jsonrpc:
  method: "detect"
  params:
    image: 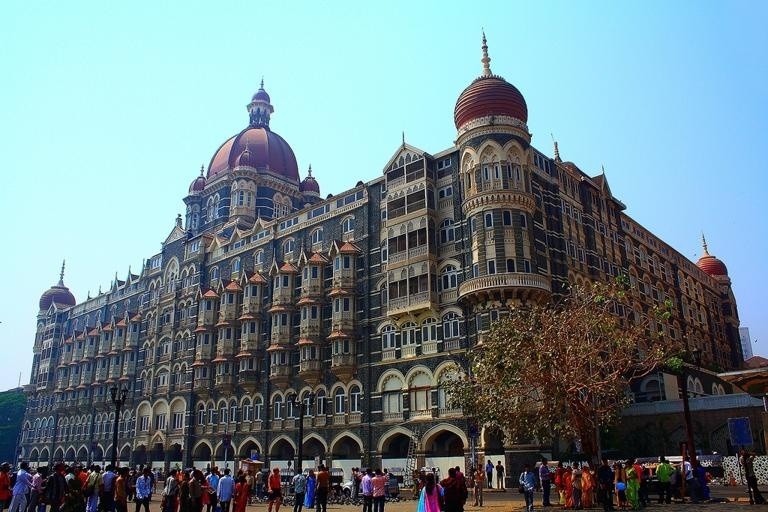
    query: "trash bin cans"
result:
[404,428,420,486]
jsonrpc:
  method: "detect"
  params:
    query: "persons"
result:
[739,447,763,505]
[349,467,390,512]
[412,466,468,512]
[517,456,711,512]
[472,464,486,506]
[485,460,505,490]
[1,459,329,512]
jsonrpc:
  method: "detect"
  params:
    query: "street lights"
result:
[658,343,702,473]
[286,390,317,476]
[109,380,130,469]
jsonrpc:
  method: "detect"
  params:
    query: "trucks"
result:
[503,449,563,490]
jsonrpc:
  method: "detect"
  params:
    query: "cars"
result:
[263,466,408,502]
